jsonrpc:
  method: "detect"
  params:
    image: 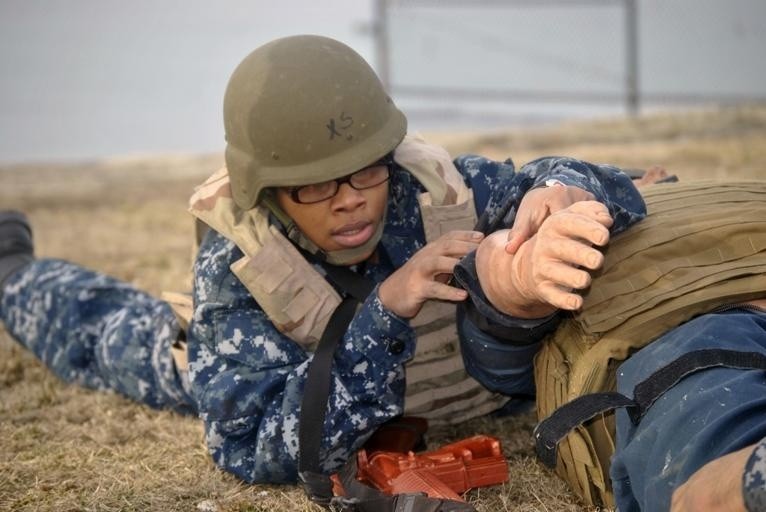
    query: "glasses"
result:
[282,154,393,204]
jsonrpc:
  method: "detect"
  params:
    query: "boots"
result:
[0,210,34,282]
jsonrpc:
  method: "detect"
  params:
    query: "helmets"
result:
[223,35,407,210]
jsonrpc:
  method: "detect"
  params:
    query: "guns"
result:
[329,435,510,503]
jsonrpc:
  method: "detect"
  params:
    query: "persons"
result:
[452,155,766,512]
[0,32,648,486]
[670,436,766,512]
